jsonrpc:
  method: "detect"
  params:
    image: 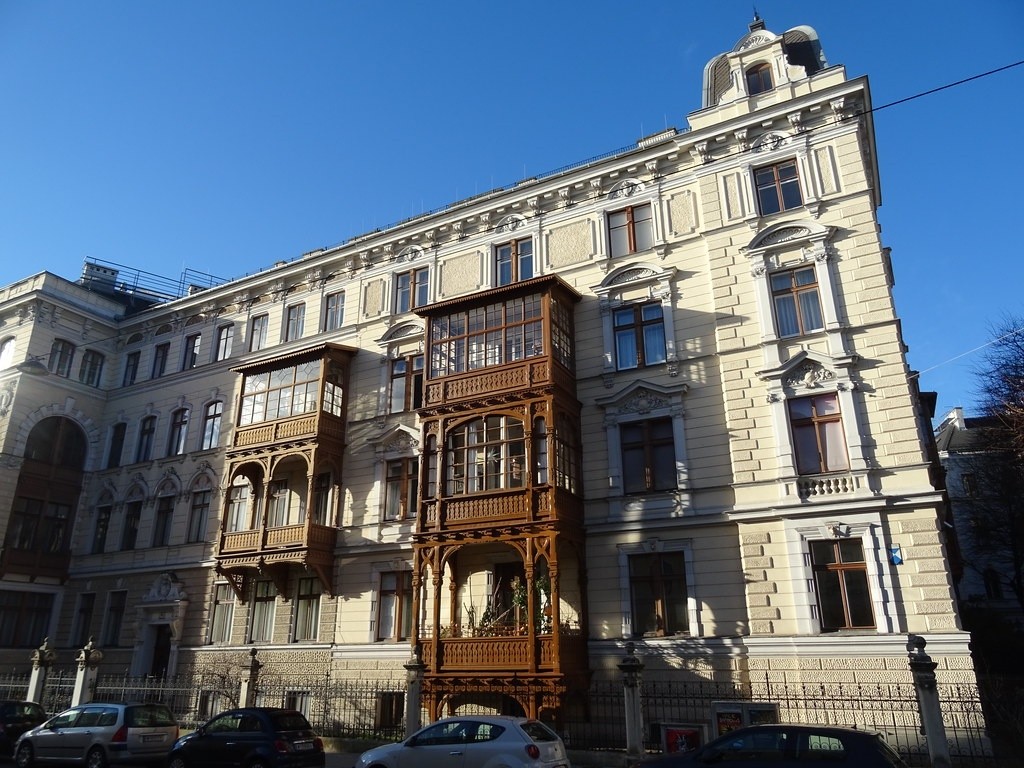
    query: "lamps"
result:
[17,354,49,375]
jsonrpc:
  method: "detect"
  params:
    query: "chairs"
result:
[239,716,257,730]
[466,725,478,739]
[78,713,102,726]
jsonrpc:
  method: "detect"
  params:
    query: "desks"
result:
[453,472,483,495]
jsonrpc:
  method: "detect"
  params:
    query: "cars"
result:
[629,721,908,768]
[0,699,49,768]
[167,706,326,767]
[13,700,179,768]
[356,715,571,768]
